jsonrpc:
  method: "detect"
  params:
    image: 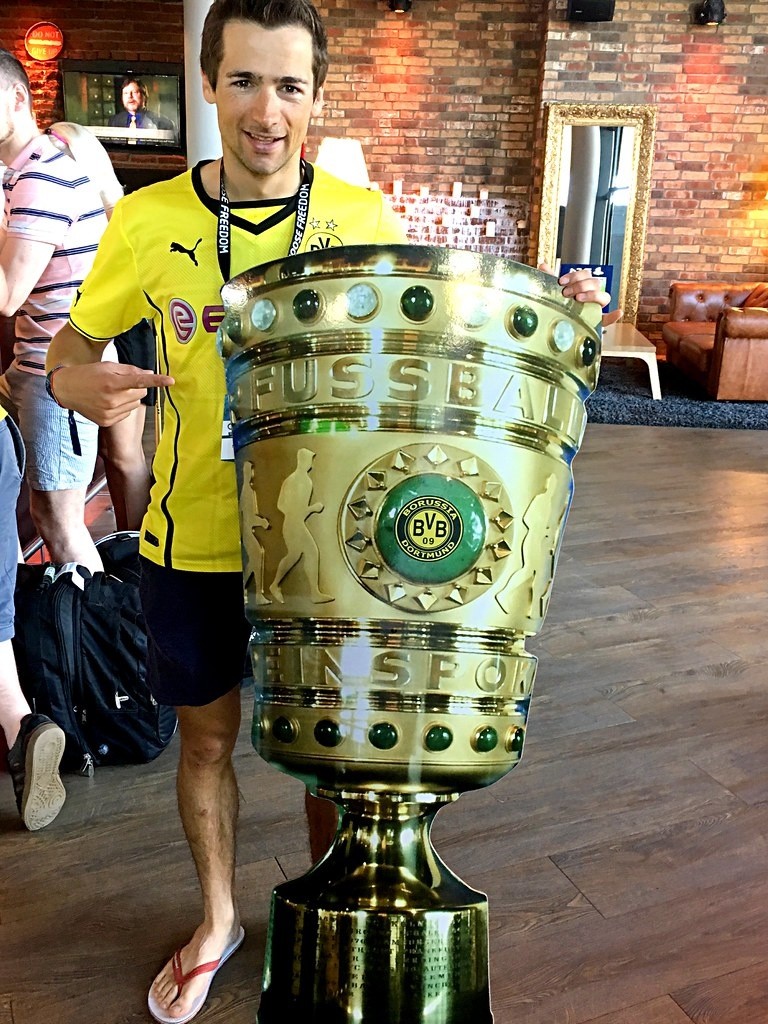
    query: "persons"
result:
[0,52,161,830]
[45,0,610,1024]
[111,75,174,145]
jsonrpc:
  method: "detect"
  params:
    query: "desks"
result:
[600,322,662,401]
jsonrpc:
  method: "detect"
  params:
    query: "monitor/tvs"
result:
[58,58,187,155]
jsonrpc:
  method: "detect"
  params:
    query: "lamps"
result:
[391,0,409,13]
[701,0,727,26]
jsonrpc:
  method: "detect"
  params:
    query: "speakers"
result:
[565,0,614,23]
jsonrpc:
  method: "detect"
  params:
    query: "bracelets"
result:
[45,365,82,457]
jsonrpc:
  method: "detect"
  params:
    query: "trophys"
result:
[221,243,604,1024]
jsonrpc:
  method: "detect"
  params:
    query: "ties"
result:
[129,117,136,128]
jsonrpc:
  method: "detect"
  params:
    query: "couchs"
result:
[663,281,768,403]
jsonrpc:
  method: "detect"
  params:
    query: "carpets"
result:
[584,356,768,431]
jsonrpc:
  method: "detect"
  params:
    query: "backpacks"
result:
[11,561,179,776]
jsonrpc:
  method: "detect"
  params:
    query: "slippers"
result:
[148,926,244,1024]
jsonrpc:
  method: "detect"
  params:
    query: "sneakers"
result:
[8,714,66,831]
